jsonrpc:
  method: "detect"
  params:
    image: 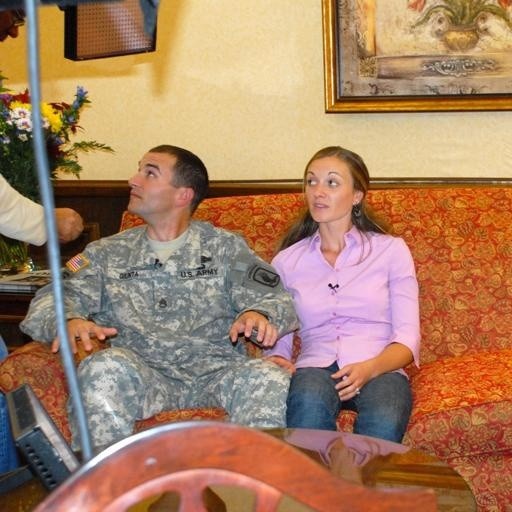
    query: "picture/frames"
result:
[322,1,512,118]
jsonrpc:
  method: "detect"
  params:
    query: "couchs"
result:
[0,181,512,511]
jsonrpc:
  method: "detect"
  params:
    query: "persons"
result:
[1,0,88,497]
[21,147,299,460]
[280,427,412,487]
[256,144,425,446]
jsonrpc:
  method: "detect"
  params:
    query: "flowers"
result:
[0,72,117,268]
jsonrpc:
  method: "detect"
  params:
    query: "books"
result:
[0,271,54,291]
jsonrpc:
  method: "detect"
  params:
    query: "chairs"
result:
[28,421,440,512]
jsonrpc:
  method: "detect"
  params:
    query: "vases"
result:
[0,234,34,277]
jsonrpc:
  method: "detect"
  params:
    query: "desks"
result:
[0,424,479,510]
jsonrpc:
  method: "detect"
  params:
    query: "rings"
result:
[354,387,361,396]
